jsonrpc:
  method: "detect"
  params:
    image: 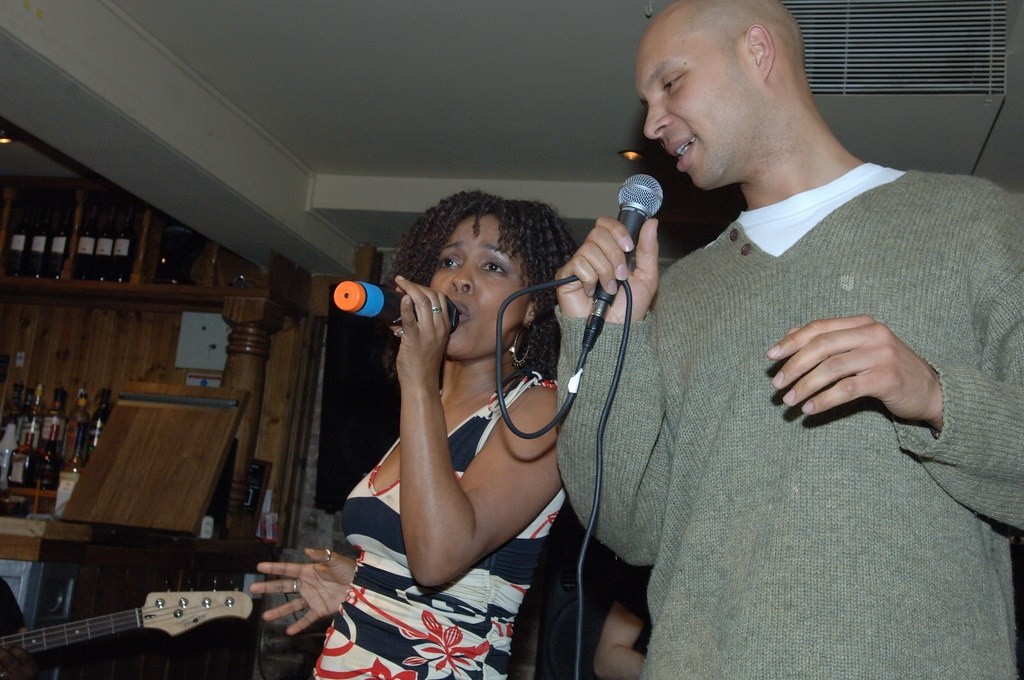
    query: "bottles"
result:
[0,380,88,486]
[8,204,135,284]
[153,217,205,285]
[87,387,112,453]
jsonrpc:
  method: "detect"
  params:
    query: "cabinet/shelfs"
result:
[0,173,312,319]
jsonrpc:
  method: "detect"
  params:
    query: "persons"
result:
[247,189,582,680]
[0,575,40,680]
[593,560,656,680]
[552,0,1024,680]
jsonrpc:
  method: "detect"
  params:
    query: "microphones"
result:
[581,174,663,349]
[334,281,459,334]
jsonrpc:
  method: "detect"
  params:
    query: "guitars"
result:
[0,575,256,662]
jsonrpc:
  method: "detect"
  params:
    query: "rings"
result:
[293,579,297,592]
[431,303,442,315]
[318,548,331,566]
[0,670,9,679]
[393,328,405,337]
